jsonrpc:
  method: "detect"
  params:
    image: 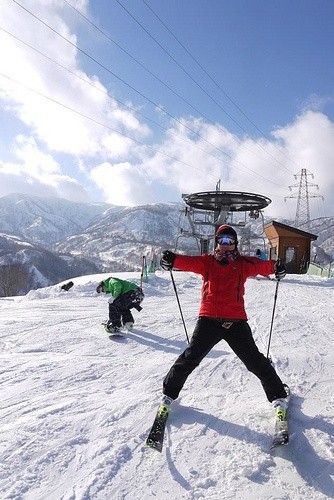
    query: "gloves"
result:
[160,251,175,270]
[274,261,286,280]
[107,296,114,303]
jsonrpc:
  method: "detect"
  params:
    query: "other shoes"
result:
[107,325,123,333]
[124,322,133,329]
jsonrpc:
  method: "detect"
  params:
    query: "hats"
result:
[215,225,236,236]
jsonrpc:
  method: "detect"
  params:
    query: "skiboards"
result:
[272,383,292,448]
[143,406,166,453]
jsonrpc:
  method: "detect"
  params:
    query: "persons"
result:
[96,276,144,334]
[156,225,287,419]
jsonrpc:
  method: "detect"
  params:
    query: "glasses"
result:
[216,235,236,244]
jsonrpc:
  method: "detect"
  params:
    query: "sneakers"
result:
[161,395,173,408]
[272,398,287,412]
